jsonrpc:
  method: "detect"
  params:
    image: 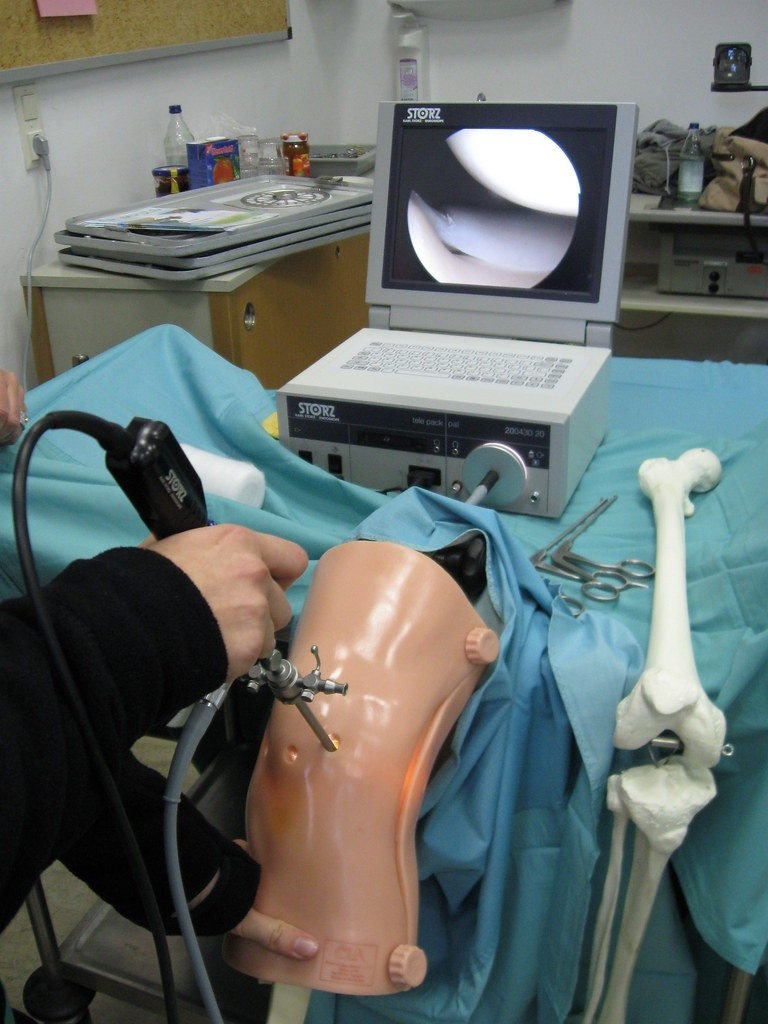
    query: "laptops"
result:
[277,104,639,423]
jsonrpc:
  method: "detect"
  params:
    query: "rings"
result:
[19,410,27,430]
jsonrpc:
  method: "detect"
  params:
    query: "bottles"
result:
[678,123,704,206]
[164,104,194,166]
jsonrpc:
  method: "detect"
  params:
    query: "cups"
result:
[236,134,286,178]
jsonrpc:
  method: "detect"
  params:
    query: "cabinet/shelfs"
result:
[619,195,768,319]
[20,170,375,391]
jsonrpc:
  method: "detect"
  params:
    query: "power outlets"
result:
[13,83,47,171]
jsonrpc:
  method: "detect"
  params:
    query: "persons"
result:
[1,369,320,1024]
